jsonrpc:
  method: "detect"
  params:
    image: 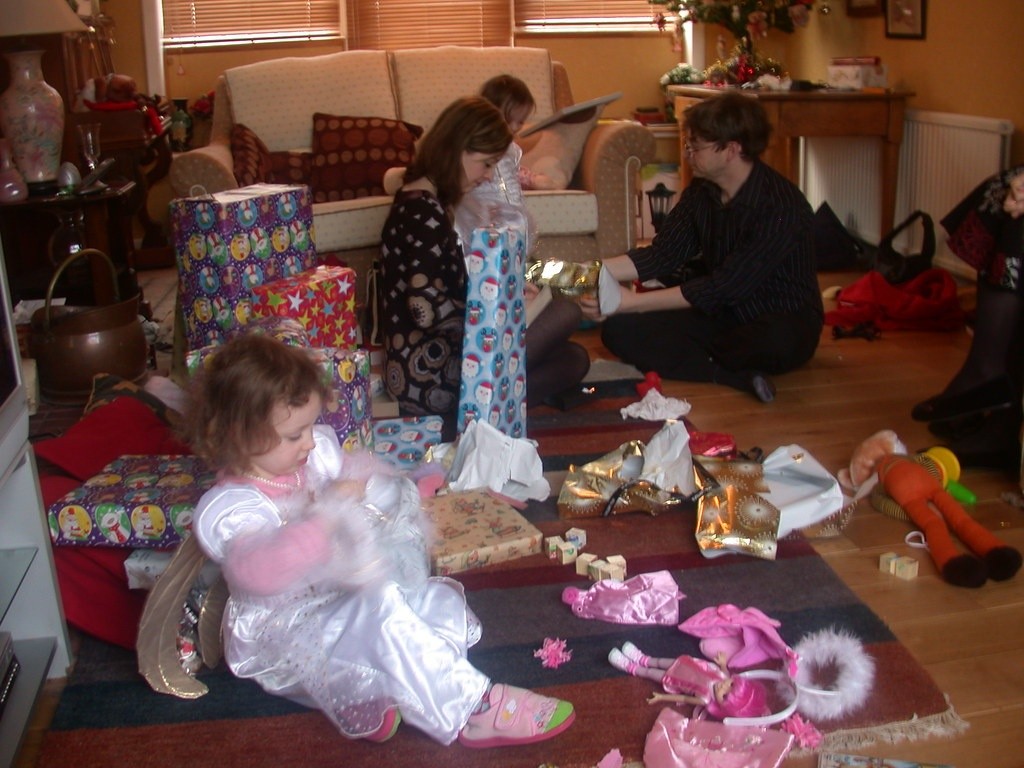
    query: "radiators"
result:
[800,108,1012,284]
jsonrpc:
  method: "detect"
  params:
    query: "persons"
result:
[911,165,1024,469]
[608,640,766,721]
[379,76,591,427]
[576,90,825,405]
[194,333,576,748]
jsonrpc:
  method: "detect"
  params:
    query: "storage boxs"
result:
[827,56,890,89]
[48,187,541,609]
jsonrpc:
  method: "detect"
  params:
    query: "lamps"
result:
[0,0,90,182]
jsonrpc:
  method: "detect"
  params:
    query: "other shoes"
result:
[458,683,577,749]
[340,698,403,744]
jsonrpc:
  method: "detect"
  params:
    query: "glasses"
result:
[684,137,718,158]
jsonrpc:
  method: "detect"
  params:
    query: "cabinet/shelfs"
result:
[0,439,76,768]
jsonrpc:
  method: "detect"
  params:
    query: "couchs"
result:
[171,47,639,300]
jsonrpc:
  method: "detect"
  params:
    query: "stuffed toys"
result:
[849,429,1024,588]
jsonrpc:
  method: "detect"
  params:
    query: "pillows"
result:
[307,112,424,202]
[231,123,312,203]
[508,104,608,190]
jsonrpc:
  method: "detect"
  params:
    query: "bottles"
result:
[169,100,192,152]
[0,140,28,202]
[0,52,65,183]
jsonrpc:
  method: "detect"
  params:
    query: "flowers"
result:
[188,91,216,146]
[649,0,818,47]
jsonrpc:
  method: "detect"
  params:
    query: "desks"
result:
[1,178,142,306]
[668,84,917,245]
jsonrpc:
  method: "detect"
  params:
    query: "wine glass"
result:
[79,123,105,187]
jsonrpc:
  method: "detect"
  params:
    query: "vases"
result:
[722,32,777,84]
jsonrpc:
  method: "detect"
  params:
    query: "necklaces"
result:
[237,467,302,492]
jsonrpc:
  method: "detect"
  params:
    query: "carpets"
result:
[30,376,964,768]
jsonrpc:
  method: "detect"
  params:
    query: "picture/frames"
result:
[885,0,929,39]
